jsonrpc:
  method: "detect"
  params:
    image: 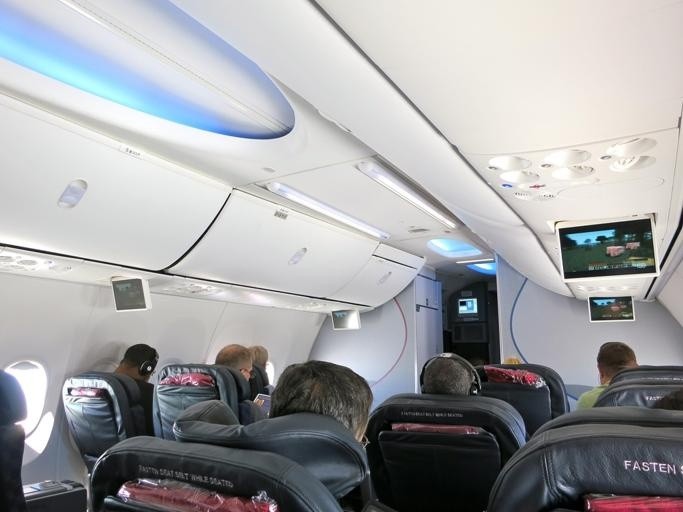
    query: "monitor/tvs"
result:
[111,277,151,312]
[556,214,661,282]
[331,310,361,330]
[588,296,635,322]
[458,299,477,314]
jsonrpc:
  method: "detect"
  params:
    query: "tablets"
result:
[254,393,270,416]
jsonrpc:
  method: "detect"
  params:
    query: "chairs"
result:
[487,407,683,512]
[89,400,371,512]
[227,365,251,400]
[592,377,683,408]
[248,363,269,395]
[152,364,255,440]
[0,370,27,512]
[62,371,146,475]
[362,393,526,512]
[474,364,570,436]
[607,366,683,385]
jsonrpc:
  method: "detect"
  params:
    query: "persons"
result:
[575,341,639,409]
[215,344,268,425]
[250,346,275,395]
[269,361,374,444]
[113,344,159,437]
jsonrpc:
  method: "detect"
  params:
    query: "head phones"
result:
[138,348,159,376]
